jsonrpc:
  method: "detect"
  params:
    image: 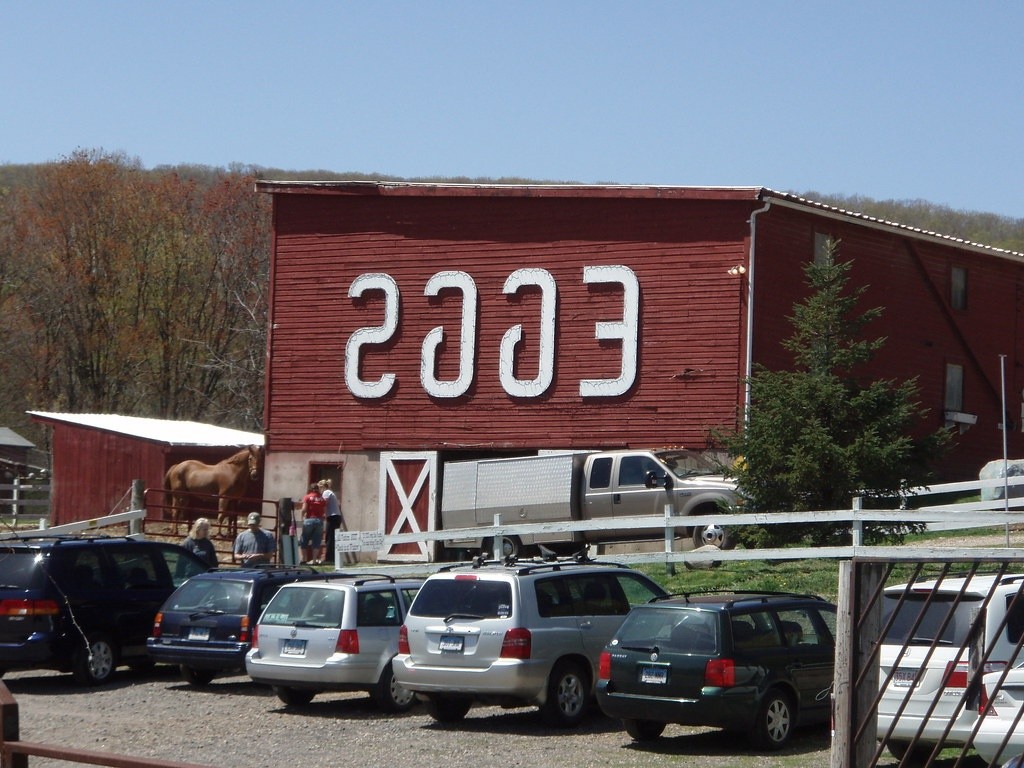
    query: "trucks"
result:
[441,442,755,564]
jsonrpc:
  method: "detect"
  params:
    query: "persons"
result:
[299,479,342,564]
[175,518,220,580]
[234,512,277,567]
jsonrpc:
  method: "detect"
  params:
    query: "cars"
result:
[971,663,1024,766]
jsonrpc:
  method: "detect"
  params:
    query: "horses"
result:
[161,446,262,539]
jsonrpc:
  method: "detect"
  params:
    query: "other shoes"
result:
[321,560,331,565]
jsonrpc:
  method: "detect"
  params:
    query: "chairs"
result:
[733,621,754,649]
[779,620,803,647]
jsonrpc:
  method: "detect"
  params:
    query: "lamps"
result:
[727,263,747,275]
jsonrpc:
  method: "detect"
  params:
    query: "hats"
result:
[309,483,319,491]
[247,512,260,526]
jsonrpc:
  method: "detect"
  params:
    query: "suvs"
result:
[592,589,838,749]
[875,571,1024,765]
[0,533,242,689]
[146,561,358,695]
[243,570,427,713]
[391,550,676,729]
